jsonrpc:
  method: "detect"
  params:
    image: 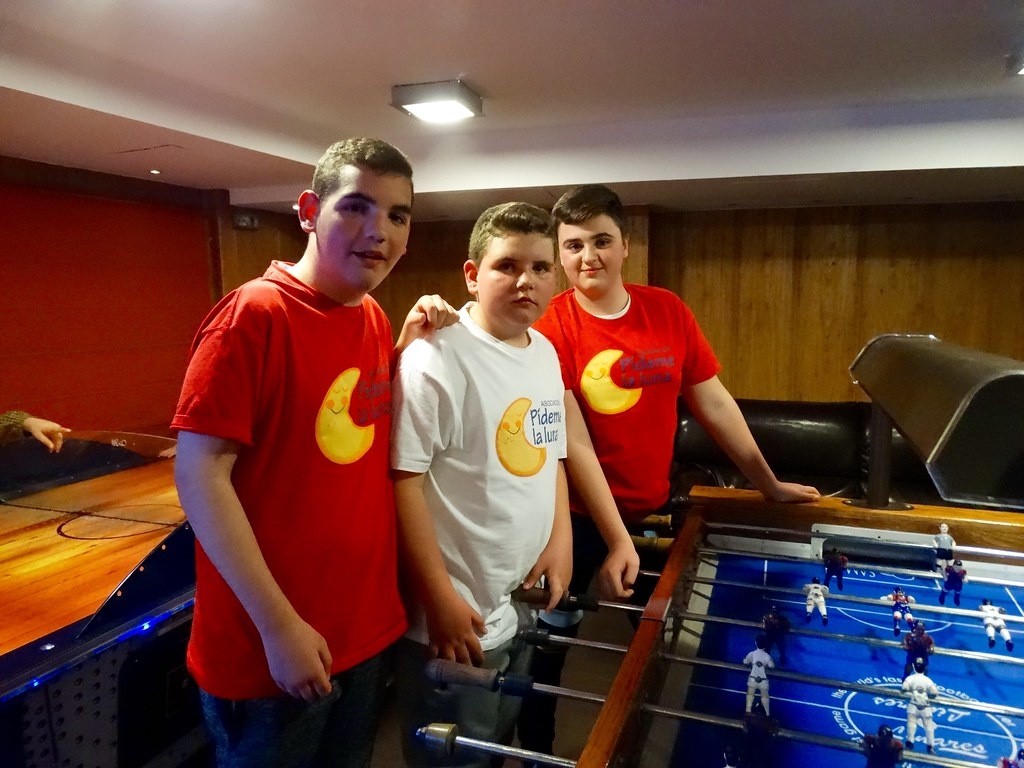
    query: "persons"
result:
[170,137,458,768]
[1,410,72,452]
[517,185,820,768]
[743,523,1024,768]
[391,204,573,768]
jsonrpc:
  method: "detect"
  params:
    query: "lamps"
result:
[388,80,484,127]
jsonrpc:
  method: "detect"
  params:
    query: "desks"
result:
[0,431,196,768]
